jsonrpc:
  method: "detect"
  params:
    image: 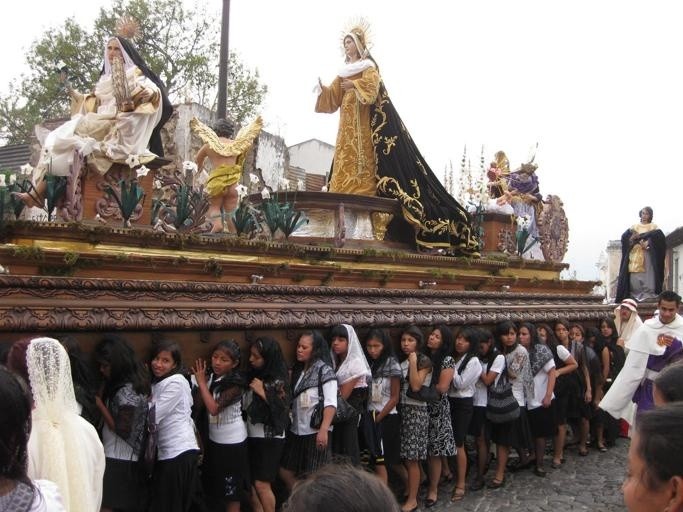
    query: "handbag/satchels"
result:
[485,383,521,423]
[406,381,440,401]
[310,363,358,429]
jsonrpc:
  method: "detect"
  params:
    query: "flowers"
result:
[0,156,307,243]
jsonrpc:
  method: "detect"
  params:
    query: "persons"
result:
[487,149,511,196]
[615,206,667,303]
[9,33,175,209]
[597,291,683,512]
[189,116,264,233]
[500,160,544,261]
[310,19,382,197]
[1,324,404,511]
[399,318,628,510]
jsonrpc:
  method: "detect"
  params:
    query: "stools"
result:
[470,211,519,255]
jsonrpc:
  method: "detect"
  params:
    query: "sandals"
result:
[399,441,609,512]
